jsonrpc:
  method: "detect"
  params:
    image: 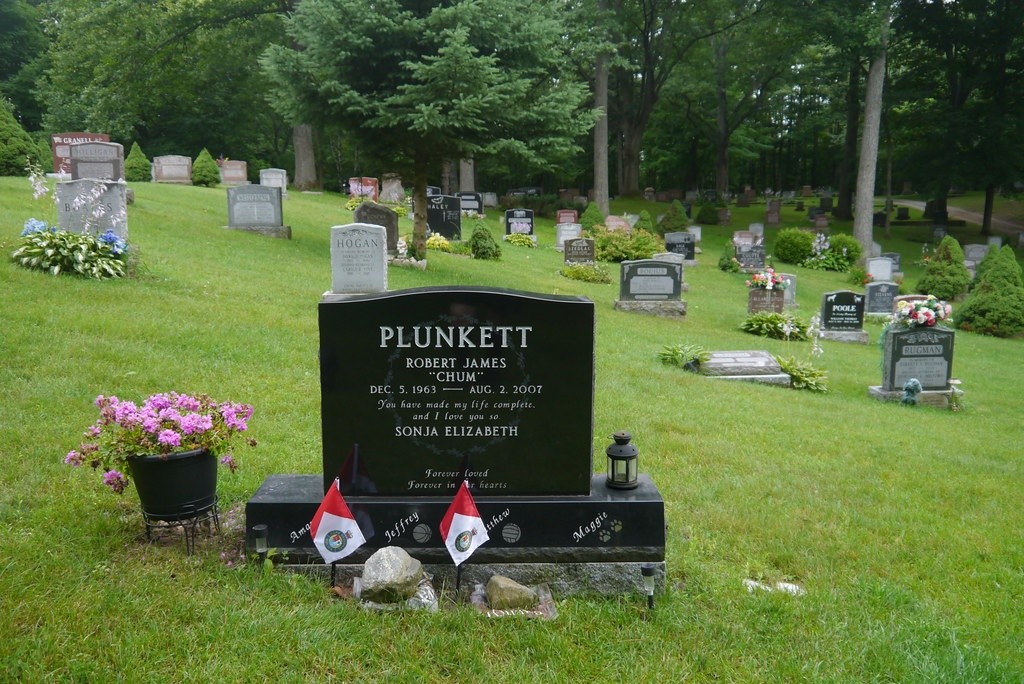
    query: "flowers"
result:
[64,391,261,493]
[9,154,129,279]
[745,266,792,291]
[731,257,745,267]
[425,232,450,250]
[810,232,830,260]
[397,235,415,259]
[882,294,955,329]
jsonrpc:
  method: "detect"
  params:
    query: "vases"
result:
[123,446,220,523]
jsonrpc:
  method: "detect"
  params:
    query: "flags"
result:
[439,478,490,567]
[310,476,368,565]
